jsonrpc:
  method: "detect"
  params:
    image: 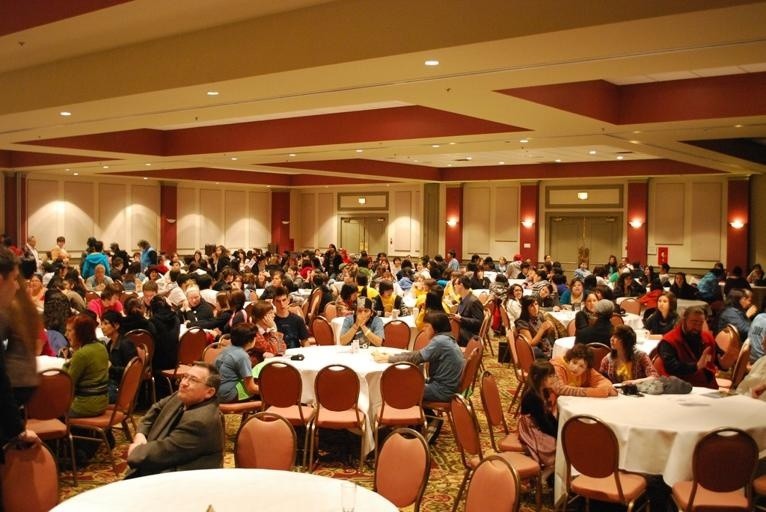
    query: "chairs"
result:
[234,412,297,471]
[451,393,542,512]
[373,361,432,466]
[71,359,142,482]
[310,361,366,471]
[465,456,520,512]
[371,428,433,512]
[671,427,760,512]
[561,415,655,512]
[479,370,535,453]
[259,361,311,473]
[1,441,60,512]
[24,370,72,480]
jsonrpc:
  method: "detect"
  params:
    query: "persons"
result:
[19,234,766,496]
[0,248,43,448]
[0,272,41,411]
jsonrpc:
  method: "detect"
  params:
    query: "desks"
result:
[556,383,766,512]
[53,468,397,512]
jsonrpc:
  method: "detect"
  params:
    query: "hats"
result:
[357,298,374,309]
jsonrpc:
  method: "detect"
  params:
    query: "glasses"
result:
[181,374,206,384]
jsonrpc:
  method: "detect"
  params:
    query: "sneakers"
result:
[536,471,549,494]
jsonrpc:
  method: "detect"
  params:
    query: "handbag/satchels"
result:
[498,341,511,363]
[614,376,693,394]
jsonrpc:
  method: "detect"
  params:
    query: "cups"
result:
[351,339,360,352]
